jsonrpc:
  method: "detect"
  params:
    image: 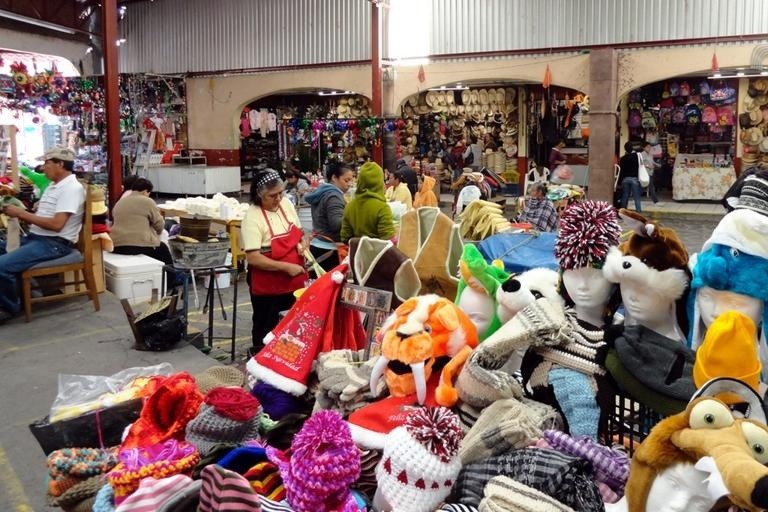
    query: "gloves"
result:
[315,347,386,409]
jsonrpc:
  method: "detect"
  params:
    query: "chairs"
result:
[22,181,102,320]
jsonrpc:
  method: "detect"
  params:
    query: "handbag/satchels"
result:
[636,152,650,188]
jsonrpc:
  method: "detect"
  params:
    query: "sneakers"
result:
[655,201,666,207]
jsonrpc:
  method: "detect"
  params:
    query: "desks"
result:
[160,262,239,367]
[158,205,244,270]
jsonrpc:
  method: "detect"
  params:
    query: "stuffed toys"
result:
[495,268,565,325]
[624,399,768,512]
[369,293,477,408]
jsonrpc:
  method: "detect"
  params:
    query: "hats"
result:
[42,438,203,512]
[244,271,345,398]
[185,386,263,460]
[35,147,75,162]
[374,404,464,511]
[738,77,768,169]
[464,384,628,510]
[400,88,517,175]
[192,410,370,512]
[693,309,763,407]
[336,95,370,118]
[90,190,115,252]
[256,259,369,368]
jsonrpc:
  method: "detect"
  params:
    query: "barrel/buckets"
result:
[179,213,213,237]
[298,207,314,230]
[203,252,234,290]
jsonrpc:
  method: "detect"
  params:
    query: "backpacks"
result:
[627,81,738,158]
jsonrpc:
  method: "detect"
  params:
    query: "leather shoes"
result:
[0,310,24,325]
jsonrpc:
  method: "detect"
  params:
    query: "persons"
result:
[278,156,438,246]
[639,142,663,207]
[561,264,613,329]
[239,168,310,360]
[698,286,765,362]
[616,141,641,213]
[549,138,568,172]
[510,182,558,231]
[459,284,495,338]
[620,280,686,349]
[0,148,174,323]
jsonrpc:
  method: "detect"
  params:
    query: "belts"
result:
[28,232,74,248]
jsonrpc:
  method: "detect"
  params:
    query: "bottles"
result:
[7,218,20,253]
